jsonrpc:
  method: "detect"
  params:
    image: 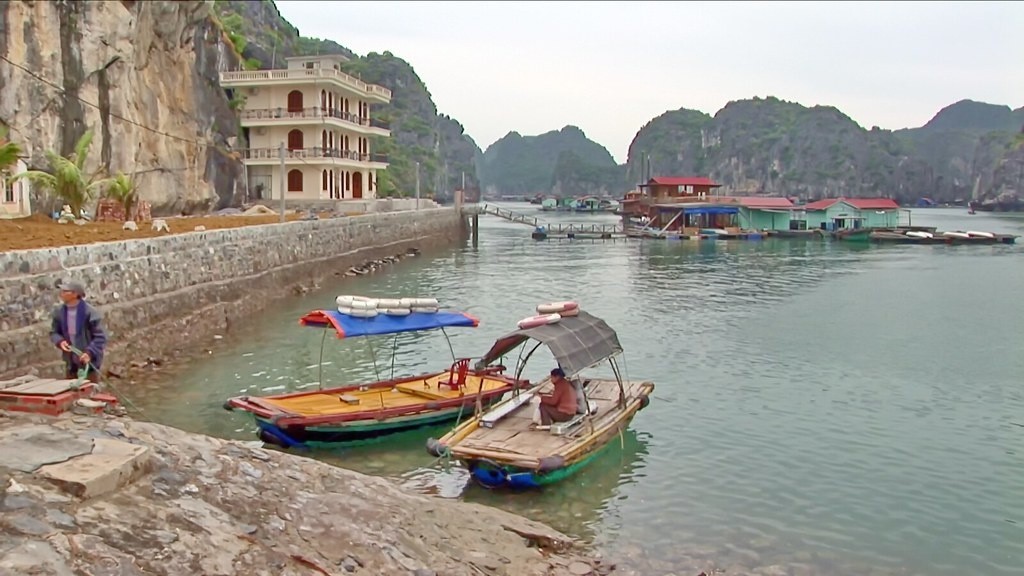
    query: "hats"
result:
[58,281,85,294]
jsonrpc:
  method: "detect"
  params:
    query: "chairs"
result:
[439,358,469,389]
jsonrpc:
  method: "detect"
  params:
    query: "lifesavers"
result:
[336,295,378,318]
[517,313,562,328]
[536,300,579,317]
[403,298,438,314]
[375,298,411,316]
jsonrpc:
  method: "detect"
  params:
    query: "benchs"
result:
[480,393,533,427]
[339,396,359,405]
[551,401,596,436]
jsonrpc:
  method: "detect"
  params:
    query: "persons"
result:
[48,280,106,384]
[536,369,578,430]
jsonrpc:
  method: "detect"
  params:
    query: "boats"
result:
[425,301,656,491]
[832,216,871,241]
[221,293,531,445]
[528,194,623,214]
